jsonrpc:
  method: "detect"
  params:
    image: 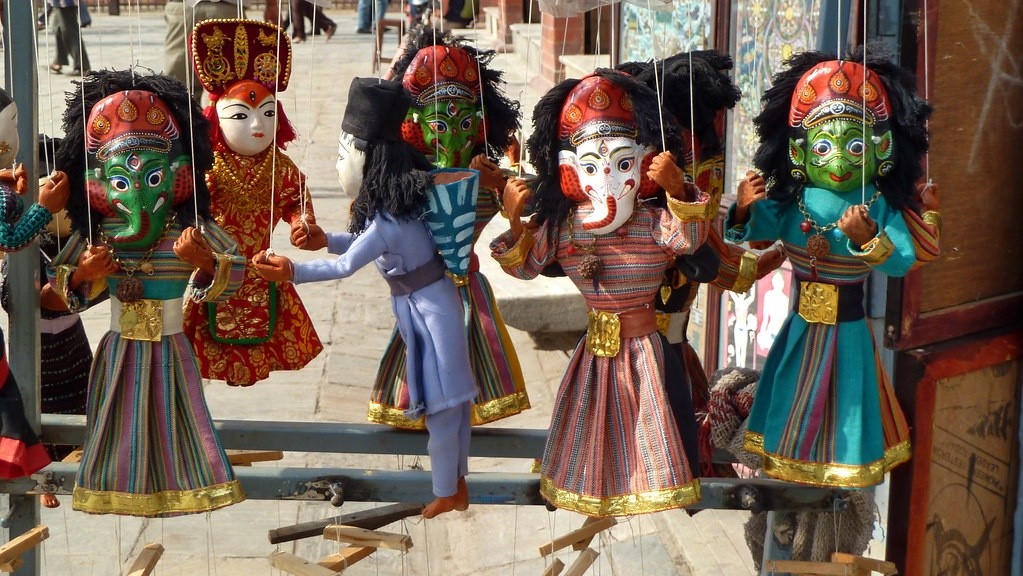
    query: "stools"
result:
[372,18,406,74]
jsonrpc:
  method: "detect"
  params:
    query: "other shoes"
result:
[374,27,392,33]
[307,30,320,35]
[357,28,371,34]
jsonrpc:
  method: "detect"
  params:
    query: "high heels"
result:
[325,26,336,40]
[292,35,306,43]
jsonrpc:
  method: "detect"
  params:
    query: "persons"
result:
[489,68,711,518]
[251,77,482,518]
[38,0,92,77]
[863,174,942,477]
[178,19,325,387]
[163,0,251,111]
[258,0,479,45]
[610,49,788,518]
[0,132,115,508]
[368,27,538,431]
[0,85,71,480]
[45,66,246,519]
[724,48,934,490]
